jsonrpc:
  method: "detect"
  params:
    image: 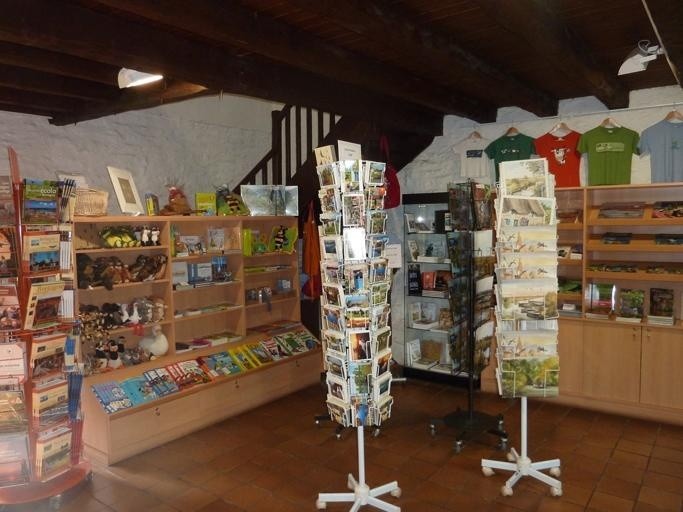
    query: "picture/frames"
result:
[107,166,145,213]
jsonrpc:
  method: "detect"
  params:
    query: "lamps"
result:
[118,67,164,90]
[618,39,662,76]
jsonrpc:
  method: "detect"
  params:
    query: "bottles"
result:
[134,226,160,246]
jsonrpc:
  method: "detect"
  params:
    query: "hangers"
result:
[446,103,683,150]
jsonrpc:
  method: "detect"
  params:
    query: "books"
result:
[406,181,496,377]
[2,175,85,486]
[92,350,242,415]
[493,158,560,396]
[314,138,393,428]
[230,327,320,370]
[174,226,301,352]
[557,201,681,325]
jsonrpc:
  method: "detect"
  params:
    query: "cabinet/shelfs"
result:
[66,215,323,466]
[402,192,480,388]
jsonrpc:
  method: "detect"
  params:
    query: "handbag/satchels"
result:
[303,220,321,277]
[385,164,400,210]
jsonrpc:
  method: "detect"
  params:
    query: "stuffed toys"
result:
[76,224,169,373]
